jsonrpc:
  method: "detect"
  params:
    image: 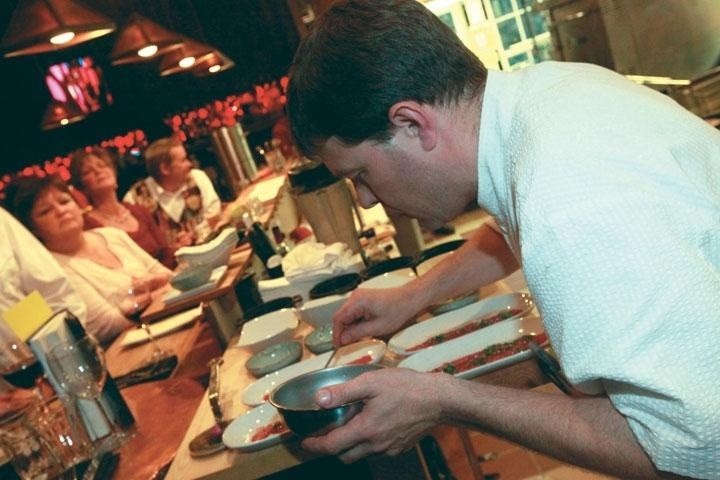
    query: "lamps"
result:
[0,0,236,79]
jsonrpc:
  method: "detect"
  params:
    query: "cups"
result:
[284,158,363,260]
[0,397,80,480]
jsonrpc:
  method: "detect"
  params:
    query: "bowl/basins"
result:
[169,264,212,292]
[174,227,239,266]
[268,364,386,435]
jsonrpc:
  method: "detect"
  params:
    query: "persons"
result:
[1,138,224,389]
[286,0,720,480]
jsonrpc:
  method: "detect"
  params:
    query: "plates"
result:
[358,266,417,295]
[416,250,459,281]
[386,292,535,357]
[160,265,228,304]
[299,289,353,327]
[395,315,551,380]
[428,291,482,313]
[303,322,336,350]
[233,308,297,348]
[120,302,203,346]
[245,343,303,376]
[222,402,290,452]
[242,339,386,405]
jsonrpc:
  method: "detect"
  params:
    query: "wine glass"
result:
[0,333,139,452]
[112,276,173,363]
